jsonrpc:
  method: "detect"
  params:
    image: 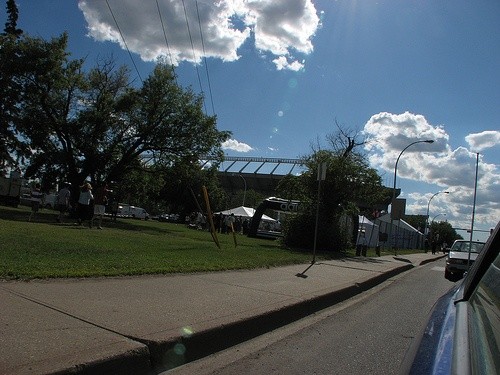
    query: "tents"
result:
[354,214,380,246]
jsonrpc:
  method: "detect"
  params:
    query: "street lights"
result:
[425,191,450,253]
[387,138,434,254]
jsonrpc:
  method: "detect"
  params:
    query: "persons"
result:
[55,182,71,224]
[159,204,283,238]
[88,180,113,231]
[28,182,44,221]
[74,182,95,226]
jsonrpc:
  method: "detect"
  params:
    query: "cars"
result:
[444,239,487,278]
